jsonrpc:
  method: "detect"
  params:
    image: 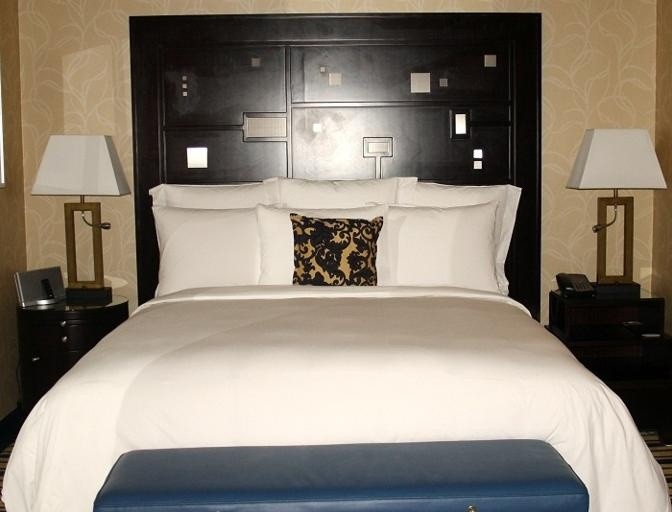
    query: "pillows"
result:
[263,176,418,209]
[413,182,522,296]
[256,203,390,285]
[290,213,383,286]
[365,198,501,295]
[149,183,266,209]
[151,203,283,299]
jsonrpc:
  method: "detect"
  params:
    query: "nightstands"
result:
[13,296,132,416]
[548,289,668,437]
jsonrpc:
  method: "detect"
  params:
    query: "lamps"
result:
[29,130,132,305]
[565,122,669,285]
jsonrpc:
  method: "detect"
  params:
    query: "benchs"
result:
[92,437,590,512]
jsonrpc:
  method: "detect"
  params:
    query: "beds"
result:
[2,290,667,511]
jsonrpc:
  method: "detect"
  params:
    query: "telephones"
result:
[556,273,595,295]
